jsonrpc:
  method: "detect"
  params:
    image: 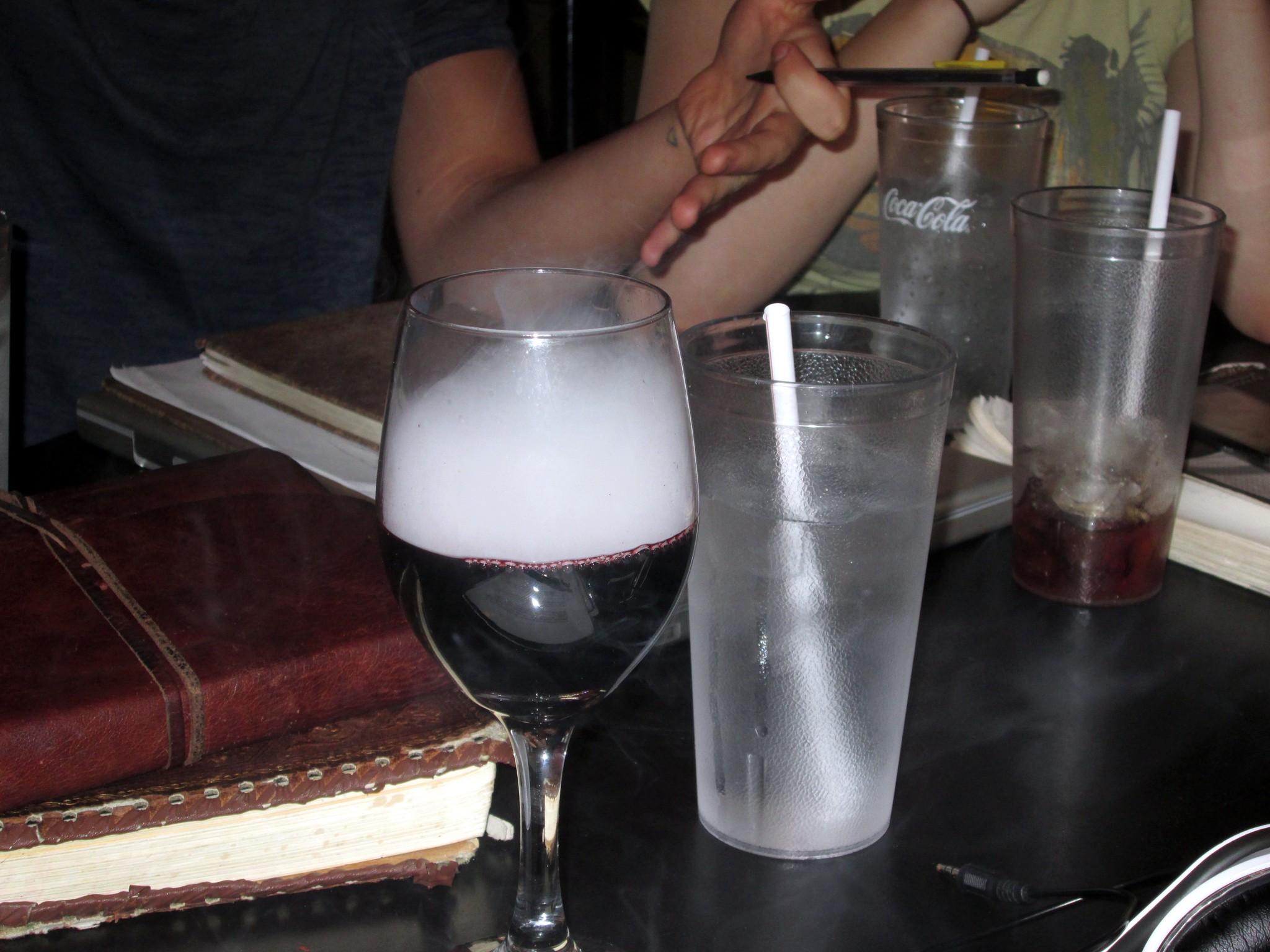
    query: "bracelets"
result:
[953,0,975,31]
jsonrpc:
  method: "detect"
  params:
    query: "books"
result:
[0,299,516,941]
[928,362,1270,599]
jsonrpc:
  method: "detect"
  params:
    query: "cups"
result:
[876,95,1048,432]
[1010,184,1227,607]
[676,313,960,856]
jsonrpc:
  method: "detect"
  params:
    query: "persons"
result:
[619,0,1270,344]
[0,0,852,460]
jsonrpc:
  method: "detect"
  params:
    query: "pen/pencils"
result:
[743,67,1049,86]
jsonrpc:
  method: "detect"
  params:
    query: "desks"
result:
[1,436,1270,952]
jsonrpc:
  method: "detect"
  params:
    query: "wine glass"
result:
[375,264,698,952]
